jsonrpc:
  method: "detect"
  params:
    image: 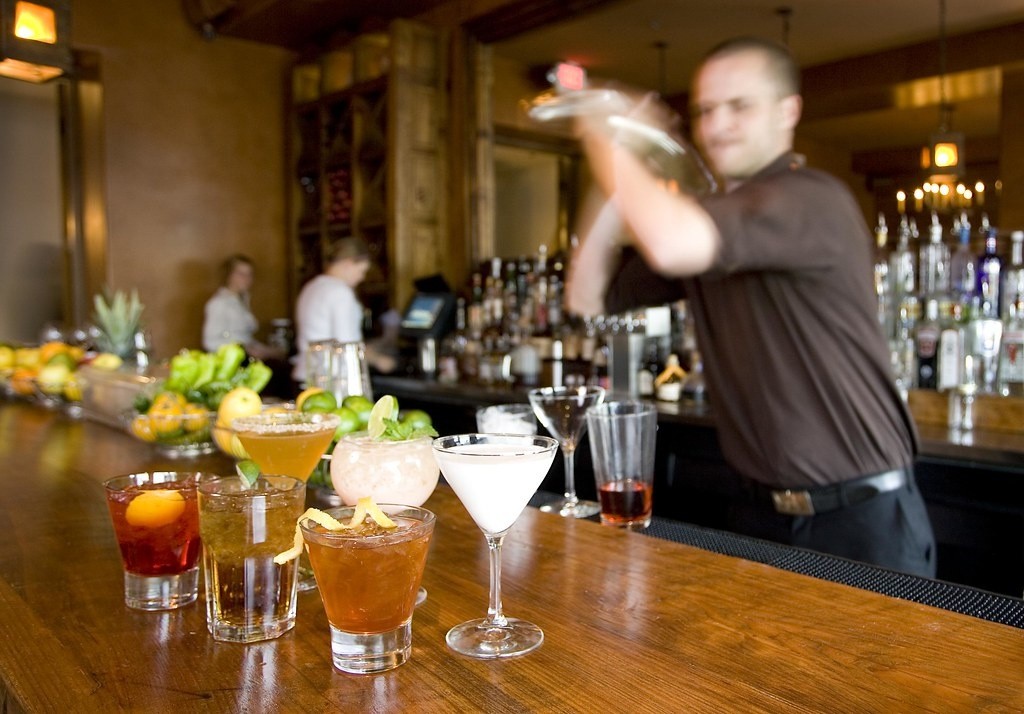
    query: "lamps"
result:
[0,0,73,87]
[920,0,967,184]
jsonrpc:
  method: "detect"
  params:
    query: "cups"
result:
[473,404,538,444]
[103,471,208,609]
[195,472,307,644]
[298,501,438,673]
[580,400,654,533]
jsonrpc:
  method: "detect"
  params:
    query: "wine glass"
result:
[432,430,561,657]
[525,384,605,519]
[329,436,440,608]
[233,411,339,590]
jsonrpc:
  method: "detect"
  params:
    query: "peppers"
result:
[131,343,271,408]
[156,429,210,447]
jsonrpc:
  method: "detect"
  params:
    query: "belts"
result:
[732,466,910,517]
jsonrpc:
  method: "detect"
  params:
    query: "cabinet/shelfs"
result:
[287,17,438,323]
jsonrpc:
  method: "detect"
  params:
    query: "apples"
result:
[211,388,327,457]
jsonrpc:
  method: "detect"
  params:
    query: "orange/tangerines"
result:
[0,339,84,398]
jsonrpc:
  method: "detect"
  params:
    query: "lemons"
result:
[235,459,262,490]
[126,489,185,526]
[302,390,435,489]
[131,391,209,442]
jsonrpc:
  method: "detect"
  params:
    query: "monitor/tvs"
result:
[399,291,459,341]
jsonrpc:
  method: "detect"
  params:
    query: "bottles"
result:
[453,190,1024,431]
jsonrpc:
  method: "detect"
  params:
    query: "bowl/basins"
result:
[123,410,269,459]
[305,428,441,508]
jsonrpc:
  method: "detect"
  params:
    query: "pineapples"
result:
[64,290,143,403]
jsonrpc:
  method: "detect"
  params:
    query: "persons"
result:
[295,236,395,408]
[199,253,277,368]
[564,36,935,579]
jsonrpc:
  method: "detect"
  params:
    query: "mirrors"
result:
[446,0,1024,254]
